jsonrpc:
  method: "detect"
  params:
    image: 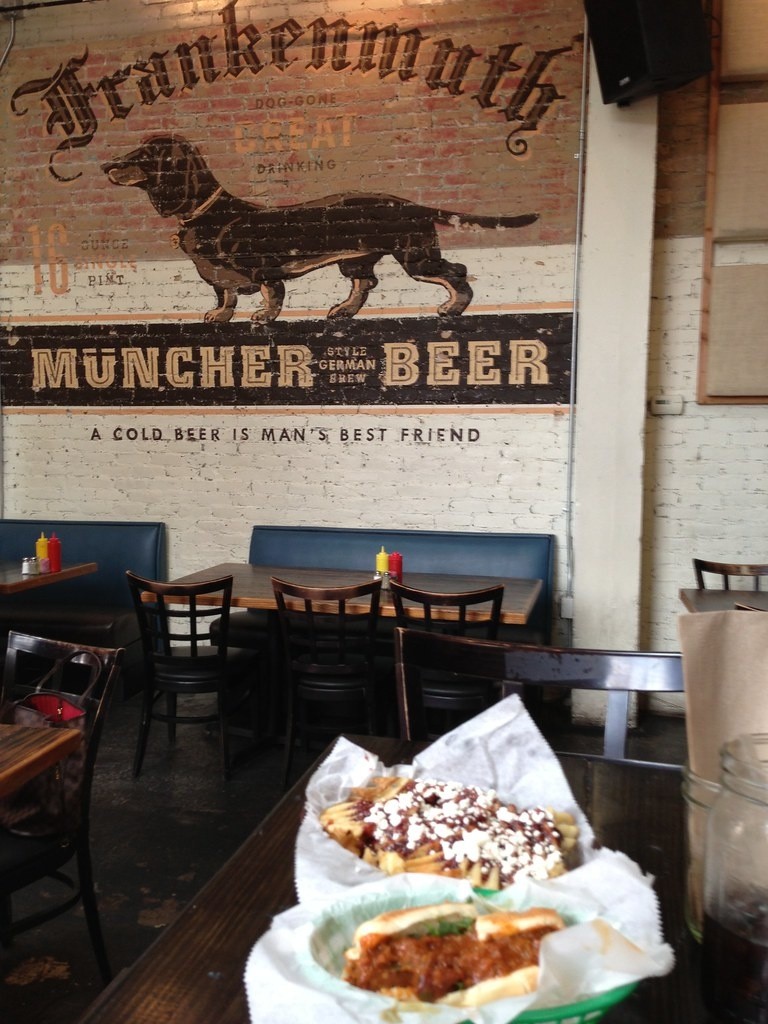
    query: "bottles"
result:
[376,545,388,577]
[373,571,382,580]
[35,531,48,561]
[47,532,61,572]
[21,557,30,574]
[30,557,39,575]
[381,573,390,589]
[388,552,402,584]
[701,732,768,1024]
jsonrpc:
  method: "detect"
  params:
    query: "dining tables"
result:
[75,733,726,1024]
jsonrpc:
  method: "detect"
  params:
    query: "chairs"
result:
[388,579,505,741]
[0,630,115,988]
[125,568,264,783]
[691,557,768,589]
[392,625,686,760]
[269,575,382,787]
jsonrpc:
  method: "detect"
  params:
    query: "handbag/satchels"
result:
[8,649,101,815]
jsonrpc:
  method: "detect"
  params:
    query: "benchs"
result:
[210,526,555,728]
[0,518,166,704]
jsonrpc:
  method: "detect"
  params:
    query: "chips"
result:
[321,777,578,889]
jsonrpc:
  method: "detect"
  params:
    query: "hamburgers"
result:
[342,904,565,1008]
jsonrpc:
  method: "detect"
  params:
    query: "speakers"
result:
[582,0,712,108]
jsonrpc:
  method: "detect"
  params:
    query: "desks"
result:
[0,558,100,594]
[680,589,768,614]
[0,723,87,799]
[141,562,545,625]
